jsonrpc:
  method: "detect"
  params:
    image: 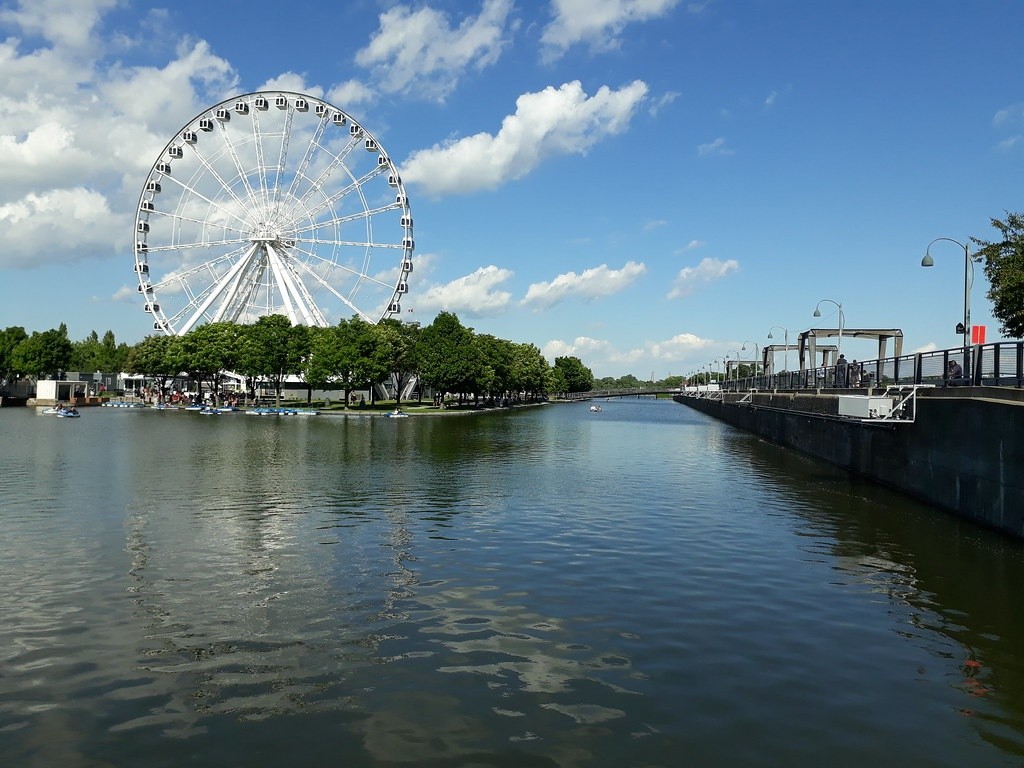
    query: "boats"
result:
[42,407,68,413]
[58,410,80,417]
[384,412,409,418]
[102,401,321,416]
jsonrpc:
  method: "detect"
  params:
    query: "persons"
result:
[590,405,602,411]
[817,354,870,388]
[52,381,260,414]
[433,393,444,406]
[939,360,962,379]
[404,391,406,400]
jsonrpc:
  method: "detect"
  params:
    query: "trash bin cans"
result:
[325,398,330,406]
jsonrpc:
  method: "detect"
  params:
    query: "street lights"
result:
[768,326,787,372]
[686,363,712,387]
[726,350,740,379]
[742,341,759,376]
[814,299,845,363]
[714,356,725,381]
[921,238,974,379]
